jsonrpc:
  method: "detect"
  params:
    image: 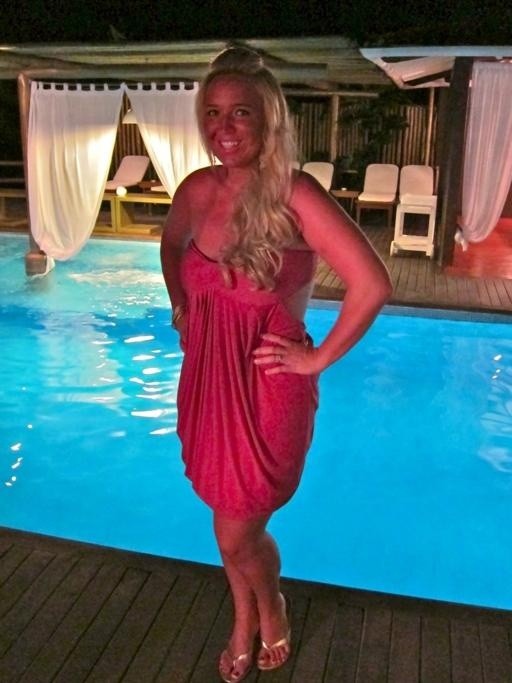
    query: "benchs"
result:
[1,188,172,236]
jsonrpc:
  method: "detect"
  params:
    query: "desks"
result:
[138,181,163,214]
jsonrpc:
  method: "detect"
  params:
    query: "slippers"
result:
[257,591,292,670]
[219,621,257,683]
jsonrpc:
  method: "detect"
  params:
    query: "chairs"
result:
[103,154,149,190]
[301,161,437,227]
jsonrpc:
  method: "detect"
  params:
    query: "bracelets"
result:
[170,301,187,329]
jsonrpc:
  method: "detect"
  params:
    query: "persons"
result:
[158,47,395,680]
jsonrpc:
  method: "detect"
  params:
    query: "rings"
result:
[272,353,282,364]
